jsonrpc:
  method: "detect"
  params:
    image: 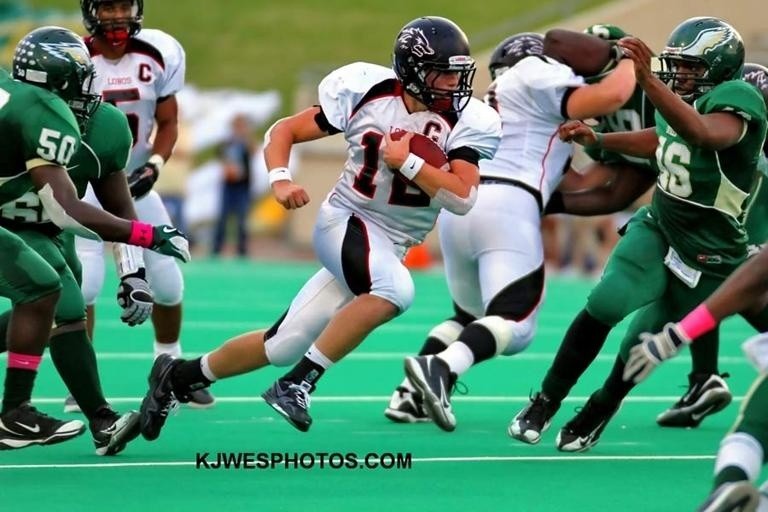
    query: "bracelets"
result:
[127,220,155,249]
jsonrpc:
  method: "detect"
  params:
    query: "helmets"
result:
[12,25,102,121]
[80,0,144,46]
[741,62,768,112]
[392,15,477,113]
[489,32,548,80]
[658,17,745,102]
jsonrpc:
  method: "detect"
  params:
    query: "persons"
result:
[62,85,157,395]
[57,0,212,413]
[0,28,196,456]
[623,240,768,512]
[140,15,767,450]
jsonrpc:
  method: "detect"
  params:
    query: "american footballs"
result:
[392,131,450,191]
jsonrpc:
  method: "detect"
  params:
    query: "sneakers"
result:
[64,387,84,412]
[0,406,86,451]
[556,389,623,453]
[140,346,214,441]
[402,352,459,431]
[695,479,761,512]
[507,389,561,445]
[657,376,732,430]
[261,378,312,433]
[93,410,141,458]
[386,384,432,422]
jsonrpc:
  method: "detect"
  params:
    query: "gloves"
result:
[130,220,191,263]
[125,154,162,201]
[116,277,154,325]
